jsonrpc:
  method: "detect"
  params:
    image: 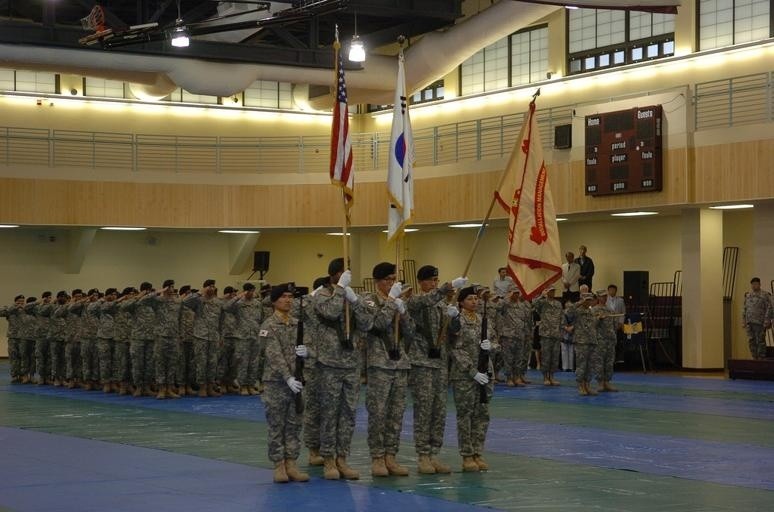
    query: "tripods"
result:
[617,313,655,373]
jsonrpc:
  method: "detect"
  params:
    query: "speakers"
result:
[254,251,270,270]
[554,125,571,149]
[624,271,649,304]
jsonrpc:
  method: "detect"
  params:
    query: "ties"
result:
[569,266,570,269]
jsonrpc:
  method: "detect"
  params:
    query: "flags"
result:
[327,42,355,227]
[385,53,416,244]
[494,101,563,302]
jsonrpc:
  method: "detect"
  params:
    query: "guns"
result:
[479,296,490,405]
[293,296,308,414]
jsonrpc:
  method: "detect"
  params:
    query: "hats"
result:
[548,286,556,293]
[457,286,478,303]
[581,292,594,301]
[271,282,296,302]
[328,257,351,277]
[417,266,438,281]
[596,290,608,297]
[509,285,520,293]
[14,278,266,307]
[372,263,396,280]
[752,278,760,284]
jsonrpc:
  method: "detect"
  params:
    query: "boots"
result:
[273,459,289,484]
[494,372,619,396]
[384,453,409,477]
[323,456,340,481]
[473,454,489,471]
[417,453,436,475]
[284,458,309,483]
[335,455,360,481]
[462,456,480,473]
[309,448,325,466]
[10,374,264,400]
[429,453,452,474]
[371,456,389,478]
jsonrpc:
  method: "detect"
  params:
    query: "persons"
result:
[449,284,502,471]
[407,264,468,474]
[305,257,374,481]
[363,262,415,478]
[259,282,319,483]
[740,277,773,360]
[300,276,332,467]
[1,278,310,400]
[477,246,626,396]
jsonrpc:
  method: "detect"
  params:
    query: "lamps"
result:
[170,1,190,52]
[345,10,367,63]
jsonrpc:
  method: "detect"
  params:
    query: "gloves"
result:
[294,344,309,359]
[478,339,492,353]
[388,282,403,300]
[286,376,303,395]
[336,270,352,289]
[473,372,489,386]
[446,304,460,319]
[394,298,405,316]
[451,277,469,289]
[310,285,324,298]
[344,286,358,304]
